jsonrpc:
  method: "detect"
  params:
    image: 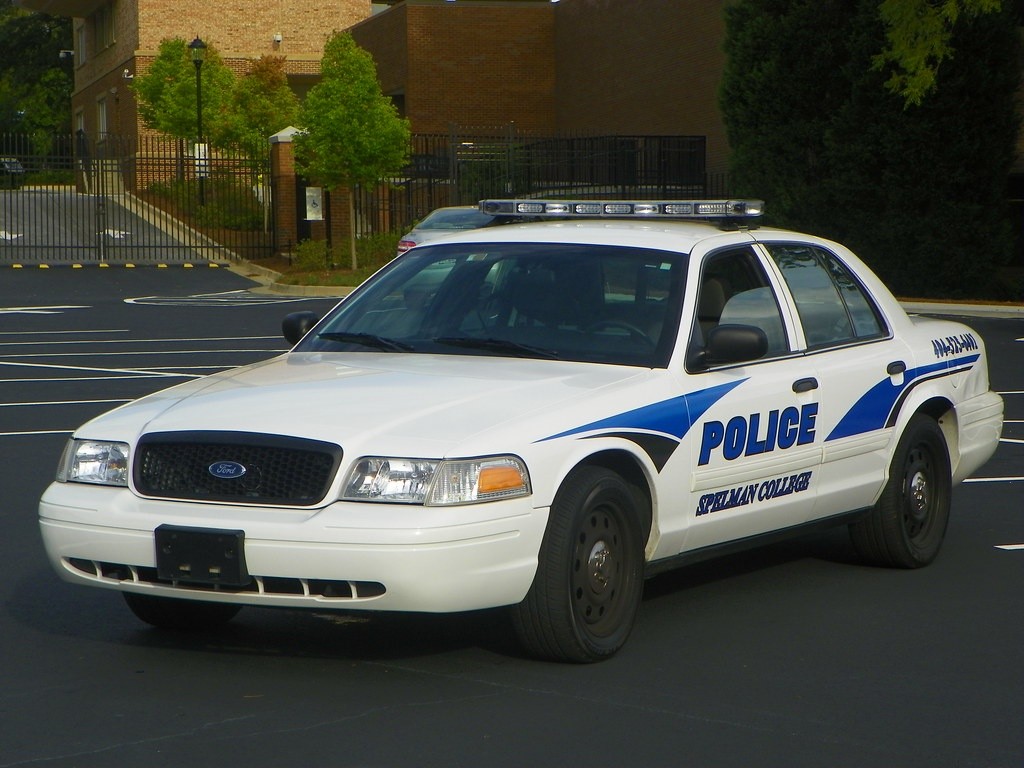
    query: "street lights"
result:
[186,36,214,203]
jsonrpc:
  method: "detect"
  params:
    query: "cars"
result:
[39,198,1007,664]
[395,205,526,308]
[0,157,26,180]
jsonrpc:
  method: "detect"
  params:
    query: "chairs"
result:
[482,259,589,346]
[646,277,727,349]
